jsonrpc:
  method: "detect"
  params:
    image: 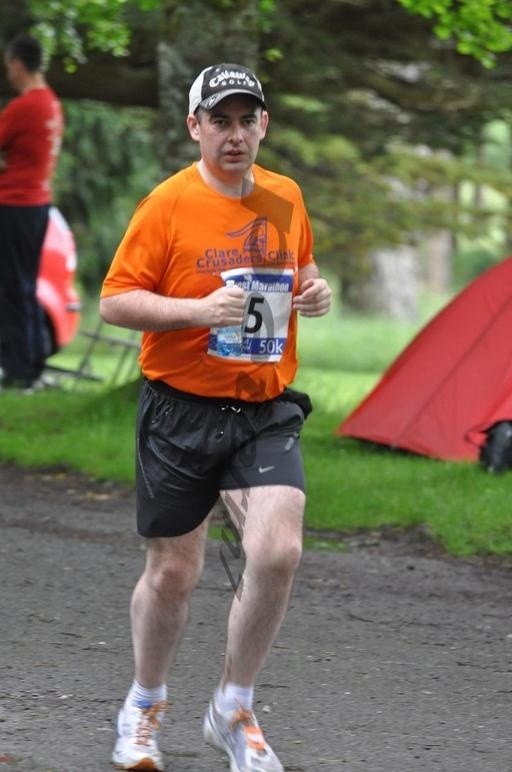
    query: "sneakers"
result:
[202,700,284,771]
[112,704,168,770]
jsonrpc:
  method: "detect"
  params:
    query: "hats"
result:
[186,60,266,115]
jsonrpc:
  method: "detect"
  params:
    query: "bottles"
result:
[214,279,243,359]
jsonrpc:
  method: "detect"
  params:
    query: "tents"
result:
[338,256,512,472]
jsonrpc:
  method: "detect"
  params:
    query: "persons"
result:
[94,60,333,771]
[0,30,65,394]
[35,205,80,359]
